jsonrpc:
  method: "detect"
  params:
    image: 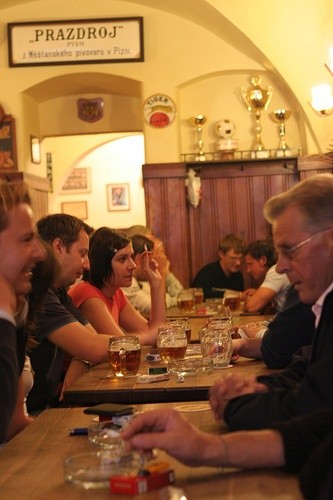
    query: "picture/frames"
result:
[0,115,18,171]
[61,201,89,220]
[60,167,92,195]
[107,183,131,212]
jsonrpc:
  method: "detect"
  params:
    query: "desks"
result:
[0,299,304,500]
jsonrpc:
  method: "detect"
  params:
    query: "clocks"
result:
[77,98,104,123]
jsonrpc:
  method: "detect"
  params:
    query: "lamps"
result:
[306,82,333,117]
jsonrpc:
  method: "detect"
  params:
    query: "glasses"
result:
[274,228,333,259]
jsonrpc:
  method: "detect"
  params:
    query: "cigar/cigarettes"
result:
[144,243,148,253]
[155,241,163,248]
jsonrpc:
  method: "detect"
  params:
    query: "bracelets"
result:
[217,434,229,468]
[72,357,93,369]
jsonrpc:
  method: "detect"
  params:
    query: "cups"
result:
[177,288,204,314]
[157,319,192,365]
[199,316,236,368]
[223,290,240,312]
[109,335,141,378]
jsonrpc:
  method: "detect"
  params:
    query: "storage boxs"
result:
[108,461,175,495]
[146,366,168,375]
[136,371,170,384]
[146,354,161,361]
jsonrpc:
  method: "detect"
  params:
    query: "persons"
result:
[0,181,62,448]
[123,224,184,313]
[68,227,167,348]
[192,235,245,303]
[210,172,333,431]
[28,214,119,411]
[121,408,333,500]
